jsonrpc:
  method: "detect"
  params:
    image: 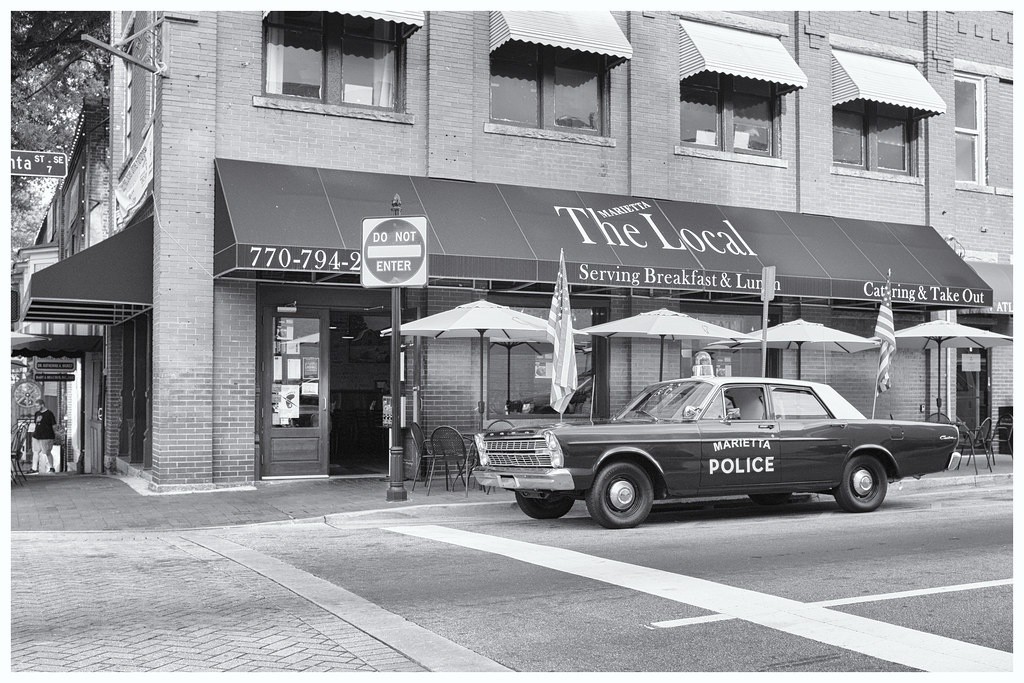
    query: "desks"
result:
[445,432,487,493]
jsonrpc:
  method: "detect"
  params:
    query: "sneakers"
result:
[48,468,55,474]
[24,469,39,475]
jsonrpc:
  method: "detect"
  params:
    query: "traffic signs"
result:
[11,150,67,178]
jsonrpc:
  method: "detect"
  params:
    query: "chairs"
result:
[11,424,27,486]
[927,414,1013,475]
[409,420,515,497]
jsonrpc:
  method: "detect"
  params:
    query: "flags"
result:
[544,254,576,413]
[874,278,896,397]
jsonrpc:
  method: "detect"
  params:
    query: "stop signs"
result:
[363,218,425,284]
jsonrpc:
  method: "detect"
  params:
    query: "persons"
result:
[24,399,56,474]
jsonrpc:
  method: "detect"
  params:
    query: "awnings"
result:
[19,216,153,327]
[956,262,1013,314]
[263,10,425,40]
[11,331,103,359]
[212,158,994,312]
[489,11,632,72]
[678,19,808,97]
[831,52,947,119]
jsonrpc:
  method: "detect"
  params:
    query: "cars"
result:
[472,376,961,530]
[504,367,596,415]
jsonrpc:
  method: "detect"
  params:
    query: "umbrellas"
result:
[868,319,1013,425]
[708,317,879,419]
[379,300,589,431]
[578,308,765,410]
